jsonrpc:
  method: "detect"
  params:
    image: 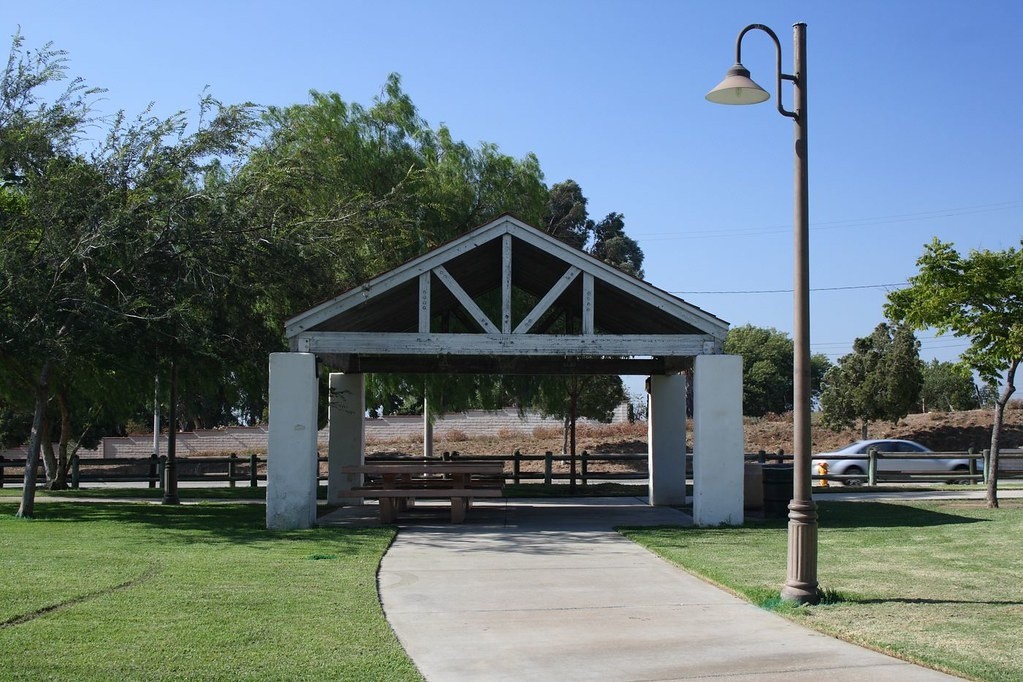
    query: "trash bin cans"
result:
[761,463,793,519]
[744,463,764,511]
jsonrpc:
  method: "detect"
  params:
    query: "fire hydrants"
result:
[818,463,830,487]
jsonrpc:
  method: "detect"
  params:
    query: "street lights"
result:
[702,23,821,605]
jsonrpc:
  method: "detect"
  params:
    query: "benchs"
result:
[345,460,506,524]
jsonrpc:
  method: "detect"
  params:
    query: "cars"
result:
[814,438,987,485]
